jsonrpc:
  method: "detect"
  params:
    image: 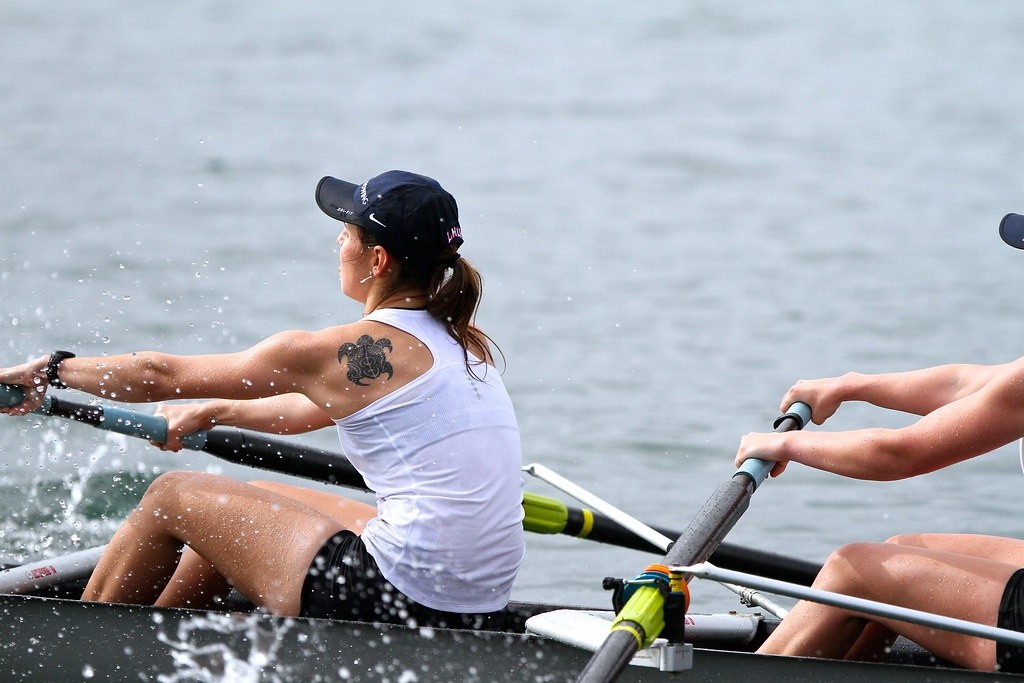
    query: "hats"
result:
[999,213,1024,251]
[314,170,464,277]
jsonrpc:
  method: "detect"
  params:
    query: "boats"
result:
[0,557,1024,683]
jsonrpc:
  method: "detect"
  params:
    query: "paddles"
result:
[0,376,821,582]
[575,397,814,683]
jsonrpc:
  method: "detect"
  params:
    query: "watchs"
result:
[47,350,75,389]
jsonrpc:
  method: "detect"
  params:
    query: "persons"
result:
[0,169,526,634]
[734,213,1024,673]
